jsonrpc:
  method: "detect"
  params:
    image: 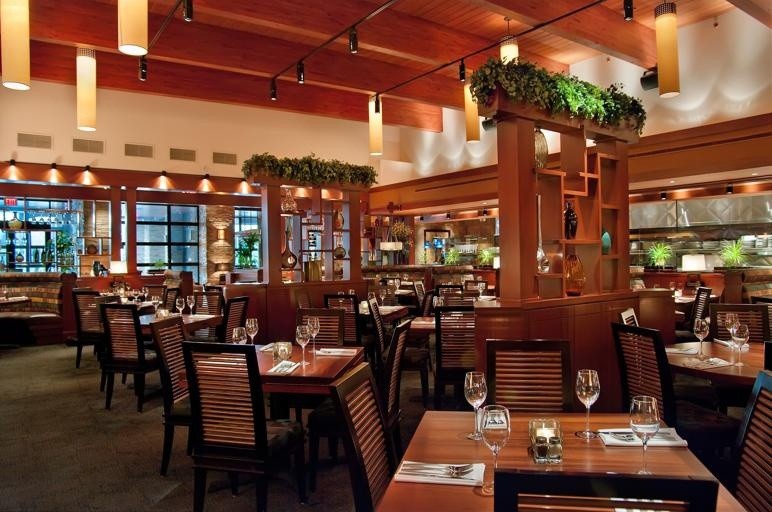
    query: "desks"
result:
[1,298,31,306]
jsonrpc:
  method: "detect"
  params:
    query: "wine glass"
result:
[694,311,749,370]
[463,368,661,496]
[653,281,685,297]
[101,282,196,322]
[231,317,321,368]
[337,271,487,322]
[31,211,75,224]
[3,232,28,247]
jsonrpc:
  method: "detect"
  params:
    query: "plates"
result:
[630,233,772,249]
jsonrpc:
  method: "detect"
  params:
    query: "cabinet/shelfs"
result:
[225,169,380,345]
[473,84,675,413]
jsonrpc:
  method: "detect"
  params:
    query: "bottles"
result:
[564,202,578,239]
[565,254,586,295]
[307,209,312,222]
[1,256,6,272]
[534,128,549,168]
[602,224,611,253]
[33,246,75,267]
[17,252,24,262]
[281,189,298,214]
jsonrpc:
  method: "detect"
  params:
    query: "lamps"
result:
[380,242,403,265]
[661,193,666,200]
[681,254,706,289]
[726,186,733,194]
[420,208,487,220]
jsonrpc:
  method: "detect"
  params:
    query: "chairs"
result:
[328,362,396,512]
[722,369,772,512]
[150,316,193,477]
[494,470,718,510]
[308,319,412,493]
[182,341,308,512]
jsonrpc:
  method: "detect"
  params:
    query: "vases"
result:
[8,212,22,230]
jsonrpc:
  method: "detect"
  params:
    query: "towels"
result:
[395,460,486,487]
[598,428,688,447]
[259,343,357,376]
[665,338,750,370]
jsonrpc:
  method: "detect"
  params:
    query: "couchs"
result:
[0,272,78,346]
